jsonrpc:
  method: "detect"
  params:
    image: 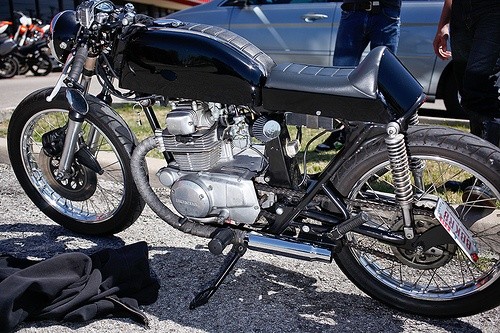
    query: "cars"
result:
[159,1,471,119]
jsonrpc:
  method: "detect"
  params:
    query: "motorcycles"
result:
[6,0,500,320]
[0,11,64,79]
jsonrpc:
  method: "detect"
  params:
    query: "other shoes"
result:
[315,142,331,153]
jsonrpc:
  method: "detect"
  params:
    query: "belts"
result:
[341,0,401,11]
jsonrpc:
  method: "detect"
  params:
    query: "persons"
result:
[315,0,401,151]
[433,0,500,208]
